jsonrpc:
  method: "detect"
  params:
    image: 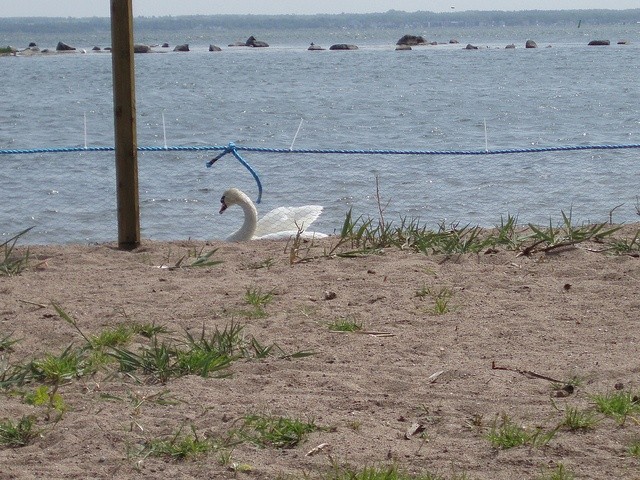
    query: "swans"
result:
[219,189,328,243]
